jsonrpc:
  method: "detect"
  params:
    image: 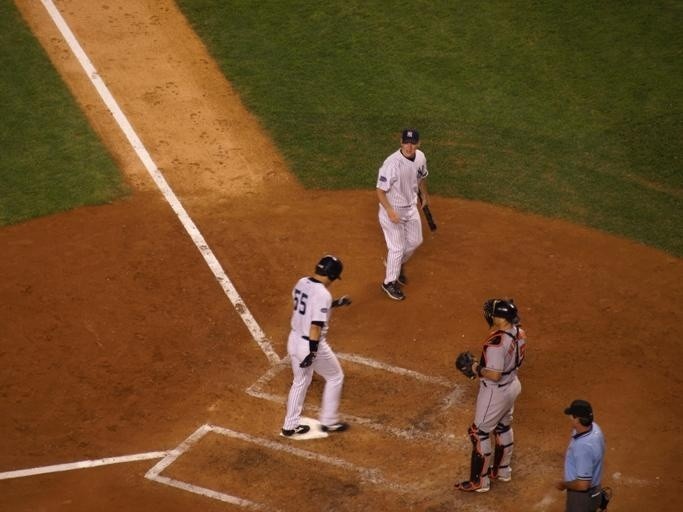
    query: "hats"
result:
[402,129,419,144]
[564,400,593,416]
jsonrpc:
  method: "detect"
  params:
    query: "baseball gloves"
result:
[456,351,478,379]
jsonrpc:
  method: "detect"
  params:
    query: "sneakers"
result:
[321,423,348,432]
[381,259,406,301]
[282,425,310,436]
[498,469,512,482]
[475,479,491,492]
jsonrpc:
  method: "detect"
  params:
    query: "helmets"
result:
[483,298,517,327]
[315,254,343,281]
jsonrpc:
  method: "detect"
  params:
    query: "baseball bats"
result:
[419,190,437,231]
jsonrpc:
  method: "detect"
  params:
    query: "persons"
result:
[454,298,527,493]
[375,129,429,301]
[282,255,351,438]
[555,400,606,512]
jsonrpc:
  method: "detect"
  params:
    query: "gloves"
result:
[337,294,351,306]
[300,352,318,368]
